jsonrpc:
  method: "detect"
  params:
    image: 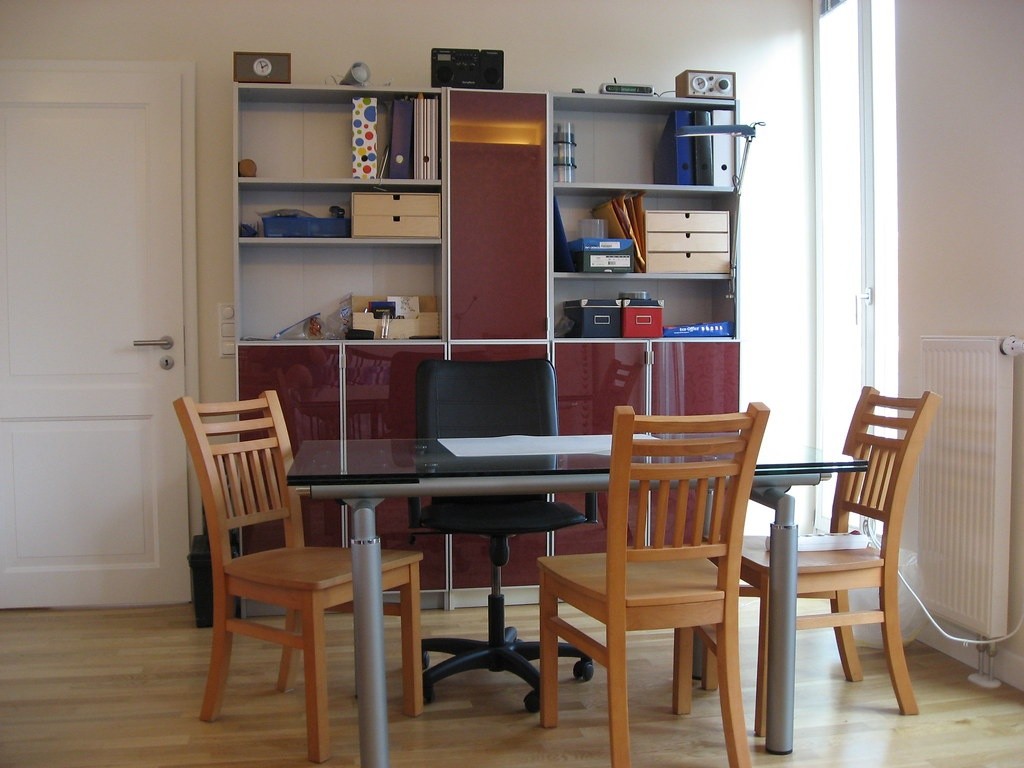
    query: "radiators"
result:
[916,334,1024,689]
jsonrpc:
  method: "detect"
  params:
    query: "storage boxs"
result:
[568,236,635,274]
[622,299,665,338]
[261,216,351,238]
[350,191,442,241]
[643,209,730,275]
[663,320,735,337]
[562,298,622,339]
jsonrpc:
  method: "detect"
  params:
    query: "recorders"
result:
[676,70,737,100]
[431,48,504,90]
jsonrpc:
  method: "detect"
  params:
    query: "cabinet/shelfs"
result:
[232,82,742,620]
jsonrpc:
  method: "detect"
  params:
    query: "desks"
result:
[286,432,869,768]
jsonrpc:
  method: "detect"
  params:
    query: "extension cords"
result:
[765,530,869,552]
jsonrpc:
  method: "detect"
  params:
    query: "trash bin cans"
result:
[186,533,241,628]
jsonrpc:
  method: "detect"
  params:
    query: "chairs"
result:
[406,355,593,714]
[701,386,945,739]
[172,390,423,764]
[536,401,771,768]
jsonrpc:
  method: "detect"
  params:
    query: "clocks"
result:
[233,51,291,84]
